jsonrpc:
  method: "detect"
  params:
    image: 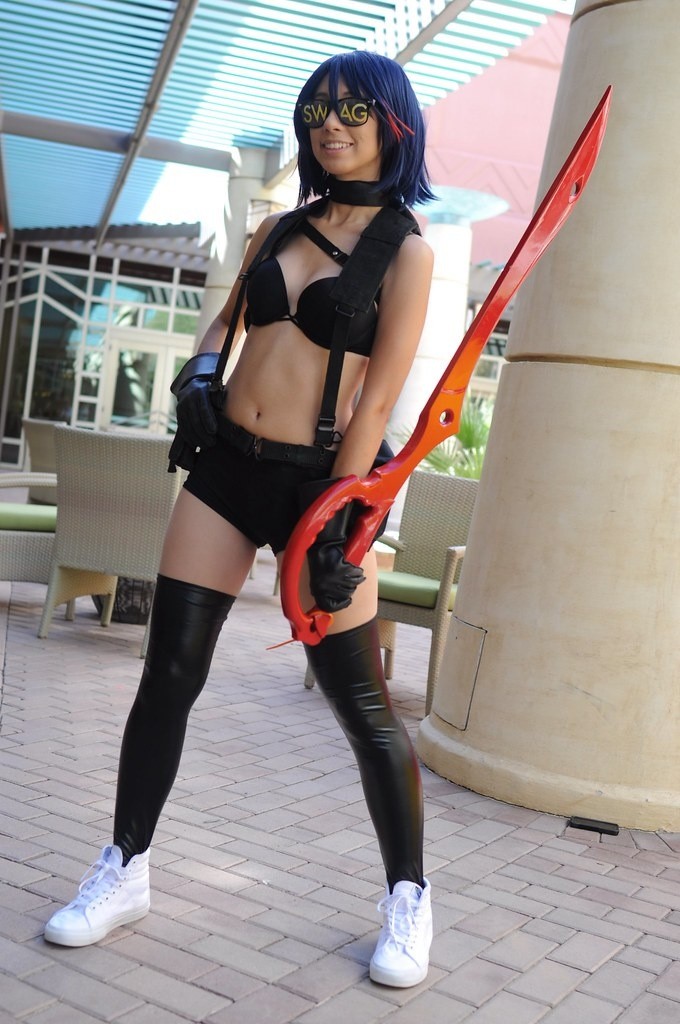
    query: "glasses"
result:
[301,99,374,127]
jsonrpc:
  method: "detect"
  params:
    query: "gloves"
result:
[170,351,226,444]
[300,479,365,613]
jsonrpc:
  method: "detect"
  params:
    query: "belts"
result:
[219,418,337,472]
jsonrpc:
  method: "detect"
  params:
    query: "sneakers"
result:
[369,878,433,986]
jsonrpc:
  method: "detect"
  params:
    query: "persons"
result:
[39,52,433,989]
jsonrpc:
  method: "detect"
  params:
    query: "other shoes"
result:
[44,847,150,946]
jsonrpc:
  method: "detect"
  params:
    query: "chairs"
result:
[0,471,57,584]
[303,471,482,716]
[37,425,190,658]
[22,417,67,506]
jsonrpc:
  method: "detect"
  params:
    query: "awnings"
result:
[1,0,572,330]
